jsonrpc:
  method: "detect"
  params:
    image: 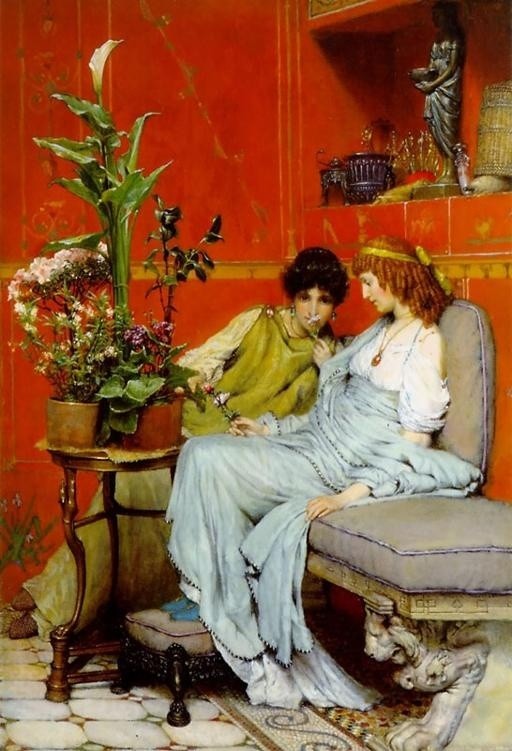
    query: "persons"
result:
[153,232,455,627]
[8,245,351,642]
[412,0,467,186]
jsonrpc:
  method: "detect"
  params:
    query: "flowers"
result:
[7,39,241,444]
[308,312,323,340]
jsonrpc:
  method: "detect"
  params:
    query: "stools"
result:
[108,610,248,728]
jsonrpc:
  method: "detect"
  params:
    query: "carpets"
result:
[201,629,433,750]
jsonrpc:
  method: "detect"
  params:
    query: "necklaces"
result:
[288,314,307,340]
[370,317,417,369]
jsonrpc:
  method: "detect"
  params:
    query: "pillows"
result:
[432,298,495,474]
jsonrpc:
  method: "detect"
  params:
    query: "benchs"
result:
[306,496,511,751]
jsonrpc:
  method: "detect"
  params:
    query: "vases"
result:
[124,401,184,454]
[45,396,99,452]
